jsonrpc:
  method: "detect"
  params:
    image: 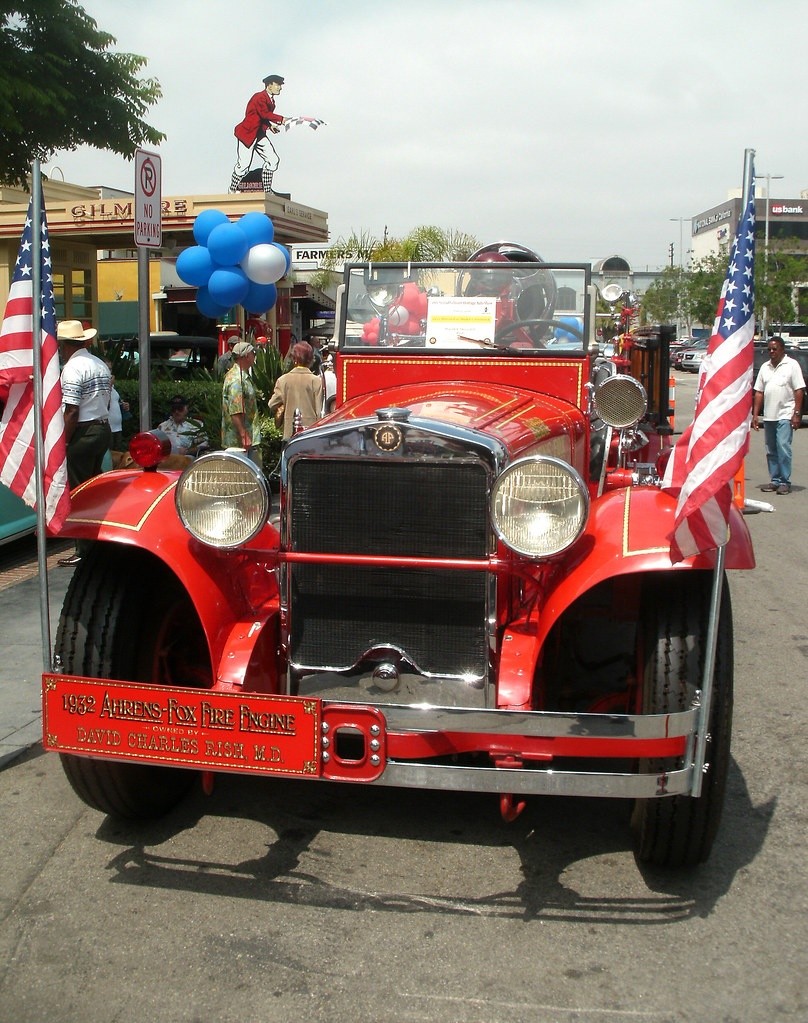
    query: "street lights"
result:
[755,170,784,341]
[669,216,693,341]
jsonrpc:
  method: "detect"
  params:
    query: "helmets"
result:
[470,252,514,296]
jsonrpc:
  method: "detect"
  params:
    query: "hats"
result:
[231,341,254,357]
[169,395,189,406]
[227,336,245,344]
[57,320,96,340]
[227,336,243,344]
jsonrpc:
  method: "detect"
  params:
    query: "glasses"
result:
[171,405,186,413]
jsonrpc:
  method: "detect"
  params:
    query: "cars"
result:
[98,332,218,384]
[668,335,808,415]
[32,261,779,875]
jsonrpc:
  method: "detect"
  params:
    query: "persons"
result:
[56,322,130,567]
[219,335,333,468]
[155,394,210,456]
[752,337,807,495]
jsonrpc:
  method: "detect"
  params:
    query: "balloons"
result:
[360,282,428,346]
[175,210,291,318]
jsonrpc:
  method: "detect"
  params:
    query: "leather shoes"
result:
[57,554,82,567]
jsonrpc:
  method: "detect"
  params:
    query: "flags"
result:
[653,164,764,570]
[0,182,70,535]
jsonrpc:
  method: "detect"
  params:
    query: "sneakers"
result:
[761,484,779,493]
[776,485,790,495]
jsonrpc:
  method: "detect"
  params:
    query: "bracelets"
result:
[793,410,804,416]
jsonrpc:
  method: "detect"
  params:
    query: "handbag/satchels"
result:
[117,398,133,438]
[274,404,284,429]
